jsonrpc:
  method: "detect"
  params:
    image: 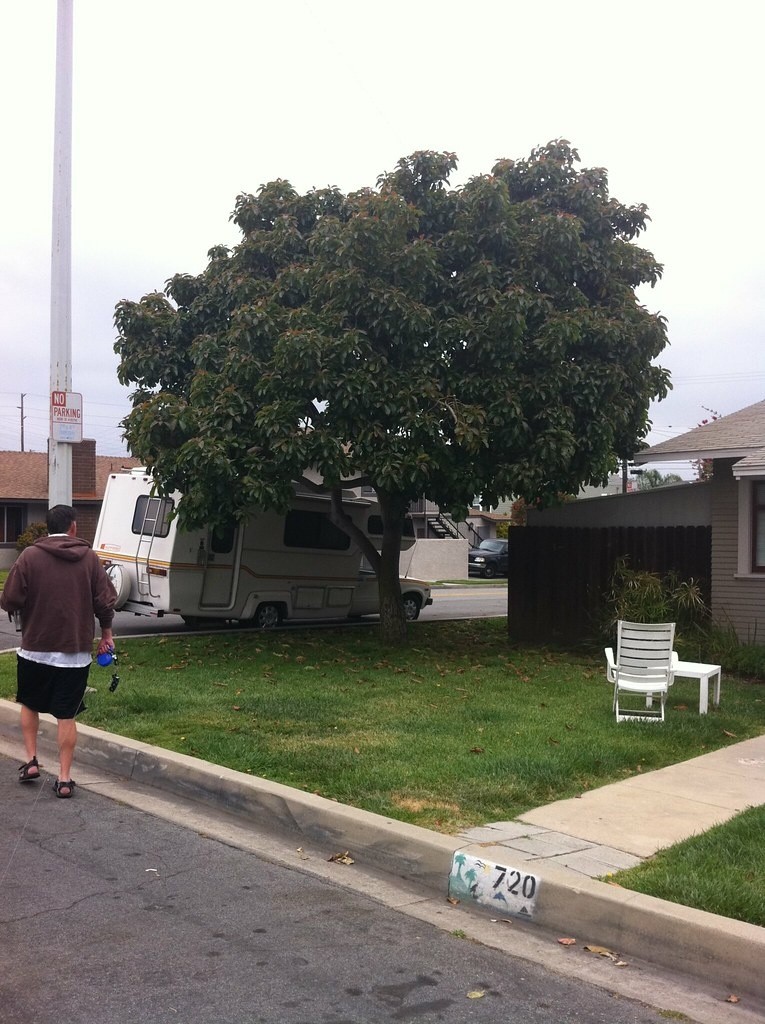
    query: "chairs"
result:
[604,620,679,724]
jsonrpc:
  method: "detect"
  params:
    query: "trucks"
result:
[91,466,433,629]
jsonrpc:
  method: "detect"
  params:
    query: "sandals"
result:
[53,778,75,798]
[18,756,40,782]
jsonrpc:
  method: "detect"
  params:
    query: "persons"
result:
[0,505,118,798]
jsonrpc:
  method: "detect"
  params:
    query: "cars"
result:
[468,539,508,579]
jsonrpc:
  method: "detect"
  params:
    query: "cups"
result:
[14,610,22,631]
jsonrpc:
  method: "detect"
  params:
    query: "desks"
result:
[646,660,722,716]
